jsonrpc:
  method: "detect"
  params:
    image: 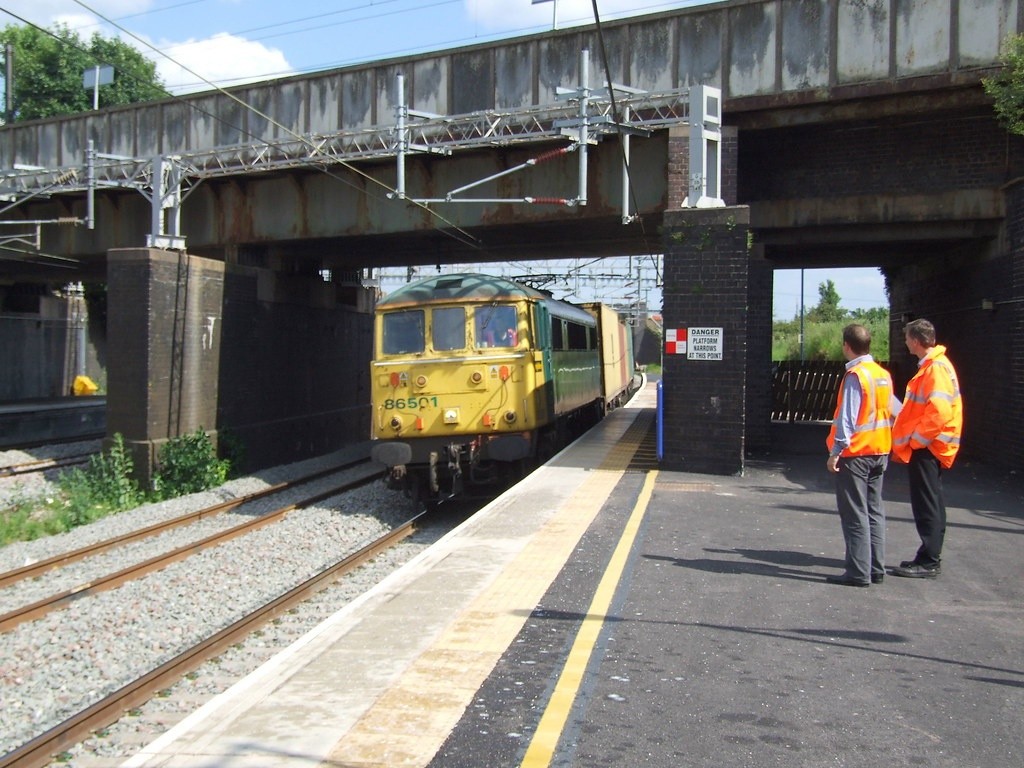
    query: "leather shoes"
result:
[894,564,937,579]
[900,560,941,574]
[871,573,884,584]
[826,575,869,588]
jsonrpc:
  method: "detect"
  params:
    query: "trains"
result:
[368,271,636,505]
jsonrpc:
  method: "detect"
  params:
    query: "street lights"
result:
[527,0,557,29]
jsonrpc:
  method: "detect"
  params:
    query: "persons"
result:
[826,324,893,589]
[486,317,518,348]
[892,319,963,578]
